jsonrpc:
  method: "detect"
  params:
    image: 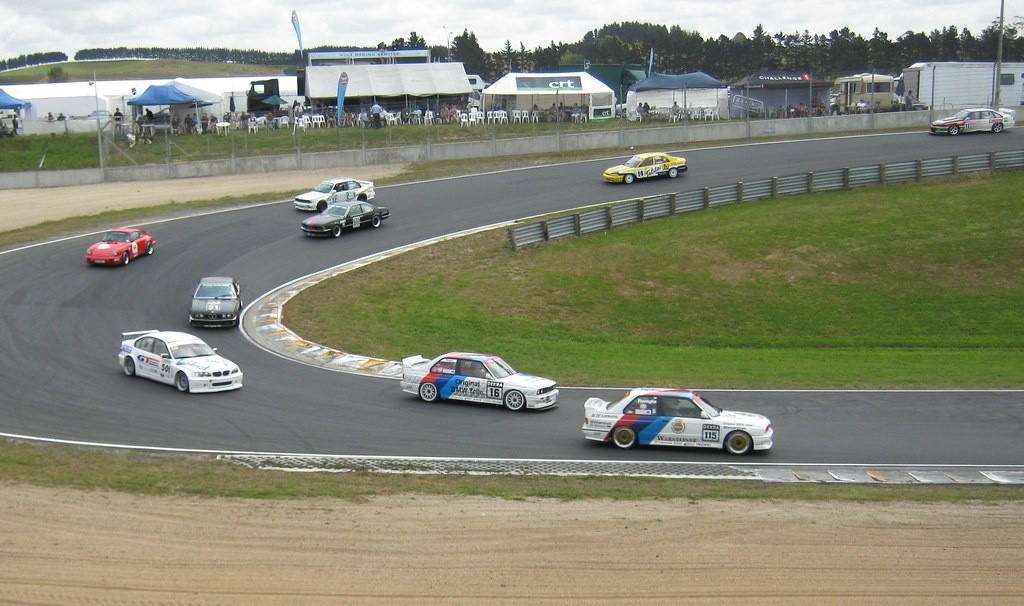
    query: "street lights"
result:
[442,25,454,59]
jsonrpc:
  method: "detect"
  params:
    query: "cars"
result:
[85,227,157,266]
[117,330,243,394]
[188,276,243,327]
[929,108,1016,136]
[399,351,561,413]
[87,109,113,121]
[294,177,376,214]
[582,387,774,457]
[300,199,391,238]
[602,152,688,185]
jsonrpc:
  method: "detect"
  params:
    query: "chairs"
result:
[635,106,720,124]
[122,108,587,134]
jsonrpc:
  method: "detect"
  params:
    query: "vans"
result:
[466,75,490,107]
[831,73,895,113]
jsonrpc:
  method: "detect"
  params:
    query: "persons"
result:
[12,118,19,134]
[858,99,871,112]
[673,102,680,121]
[533,102,588,124]
[668,399,693,416]
[292,101,388,129]
[778,94,840,118]
[47,112,66,136]
[637,102,654,122]
[114,108,124,137]
[473,363,487,376]
[172,112,218,136]
[222,108,284,131]
[401,102,467,125]
[135,108,153,123]
[494,103,500,111]
[905,90,915,109]
[338,185,343,192]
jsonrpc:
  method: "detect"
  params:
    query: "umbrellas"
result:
[262,96,289,105]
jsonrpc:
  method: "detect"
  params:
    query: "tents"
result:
[303,62,474,111]
[626,72,729,121]
[480,73,617,117]
[730,72,835,118]
[127,84,200,129]
[0,88,31,120]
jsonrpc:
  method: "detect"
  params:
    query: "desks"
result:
[216,122,230,136]
